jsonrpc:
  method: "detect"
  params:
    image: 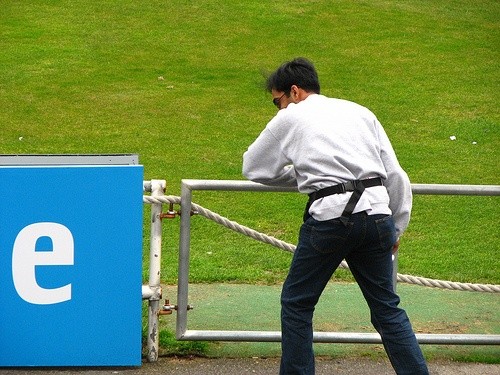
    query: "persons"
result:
[240,57,430,375]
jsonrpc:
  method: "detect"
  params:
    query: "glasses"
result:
[273,86,301,108]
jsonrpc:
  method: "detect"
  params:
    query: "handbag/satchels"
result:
[303,193,314,222]
